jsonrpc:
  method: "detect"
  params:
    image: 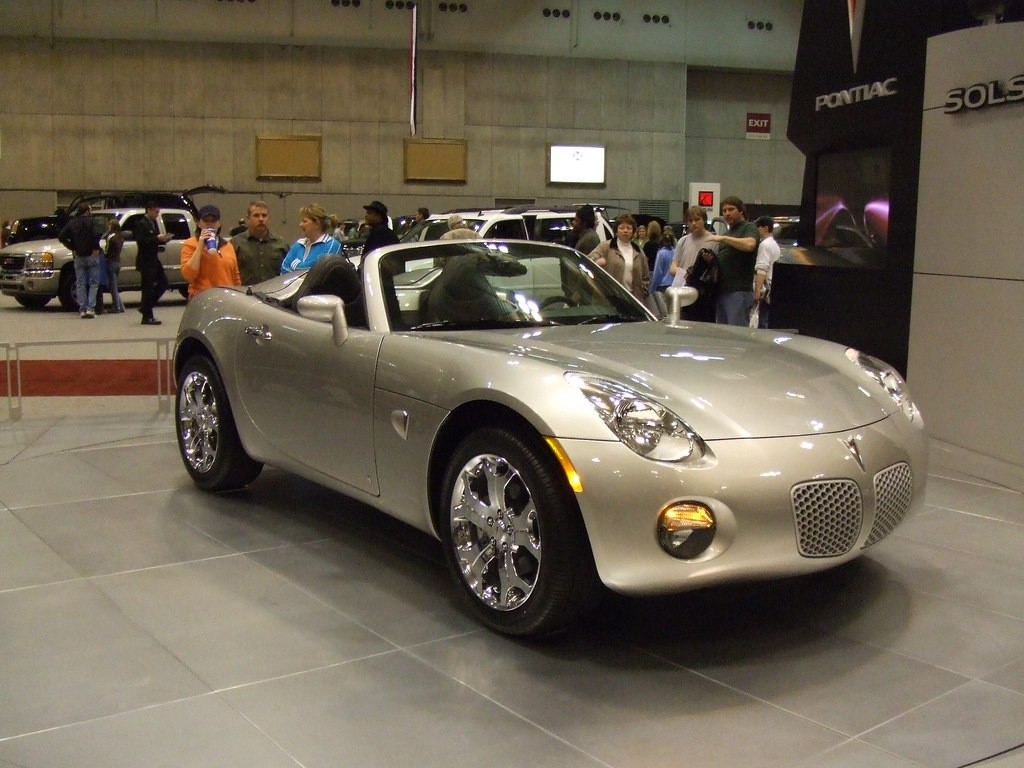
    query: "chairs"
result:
[289,254,362,330]
[425,257,515,326]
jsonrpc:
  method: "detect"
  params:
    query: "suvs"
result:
[345,202,631,273]
[8,184,228,245]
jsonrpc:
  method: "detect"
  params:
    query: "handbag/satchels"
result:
[753,274,770,301]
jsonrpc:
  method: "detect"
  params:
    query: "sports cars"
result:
[172,238,927,643]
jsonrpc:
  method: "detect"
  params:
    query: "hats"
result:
[199,205,220,220]
[363,201,388,217]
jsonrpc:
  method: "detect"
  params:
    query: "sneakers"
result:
[107,306,120,313]
[119,309,125,313]
[80,310,96,318]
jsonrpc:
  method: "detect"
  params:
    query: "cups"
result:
[206,229,216,253]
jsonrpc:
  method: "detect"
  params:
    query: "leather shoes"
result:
[137,305,143,314]
[142,317,161,325]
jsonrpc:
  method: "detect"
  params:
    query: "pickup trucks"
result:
[0,208,233,313]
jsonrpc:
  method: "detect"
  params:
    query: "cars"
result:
[612,214,800,242]
[339,215,416,235]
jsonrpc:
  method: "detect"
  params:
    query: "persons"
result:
[559,195,781,329]
[1,221,10,248]
[180,200,488,301]
[135,202,168,325]
[58,202,125,319]
